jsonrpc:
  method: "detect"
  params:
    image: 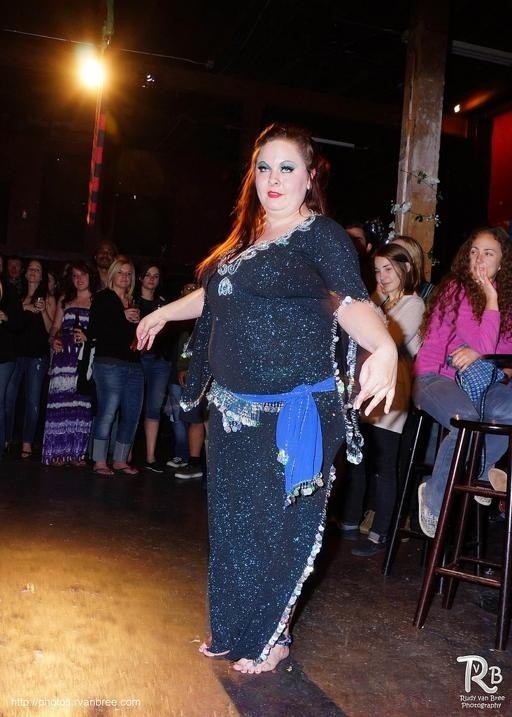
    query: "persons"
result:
[390,234,436,303]
[409,224,512,539]
[342,224,375,256]
[2,242,209,480]
[137,126,398,676]
[335,243,426,556]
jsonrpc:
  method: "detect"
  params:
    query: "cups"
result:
[128,302,139,325]
[33,296,43,302]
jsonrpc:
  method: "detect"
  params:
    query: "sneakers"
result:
[417,483,439,538]
[326,509,412,556]
[473,468,508,512]
[142,457,203,479]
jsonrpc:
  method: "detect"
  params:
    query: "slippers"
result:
[20,448,32,459]
[92,468,115,476]
[112,465,139,476]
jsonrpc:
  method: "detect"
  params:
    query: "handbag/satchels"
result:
[448,347,506,402]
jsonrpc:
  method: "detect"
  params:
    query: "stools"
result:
[381,411,449,576]
[411,419,512,652]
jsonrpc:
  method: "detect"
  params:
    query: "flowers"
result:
[425,174,442,188]
[390,200,412,215]
[388,221,395,240]
